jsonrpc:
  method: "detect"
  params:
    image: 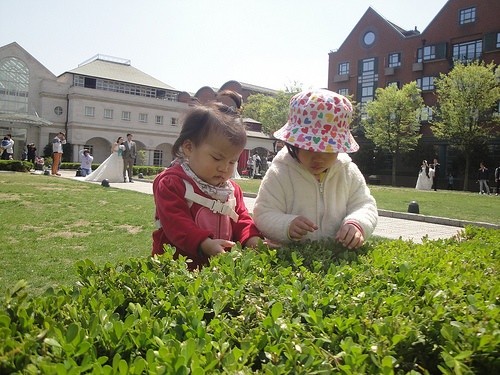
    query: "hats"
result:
[273,89,360,153]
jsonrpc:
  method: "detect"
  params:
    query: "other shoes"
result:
[129,181,134,183]
[56,174,61,176]
[52,174,57,176]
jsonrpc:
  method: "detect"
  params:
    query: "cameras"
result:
[87,150,89,153]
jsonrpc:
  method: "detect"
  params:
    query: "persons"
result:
[475,161,500,196]
[85,136,129,182]
[79,149,94,177]
[121,133,136,183]
[247,152,276,180]
[416,159,435,190]
[151,101,276,269]
[253,90,378,248]
[0,134,45,165]
[431,158,440,190]
[52,132,66,177]
[447,173,454,190]
